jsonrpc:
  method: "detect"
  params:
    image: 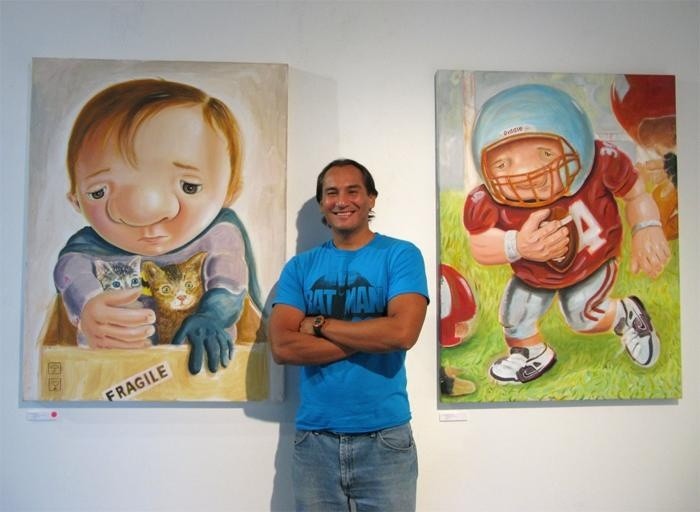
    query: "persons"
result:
[36,74,270,374]
[268,159,430,512]
[460,81,673,384]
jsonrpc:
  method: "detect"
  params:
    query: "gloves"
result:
[173,286,249,374]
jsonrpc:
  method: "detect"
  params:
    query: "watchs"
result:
[313,315,325,336]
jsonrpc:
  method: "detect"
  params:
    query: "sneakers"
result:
[614,295,660,368]
[489,341,556,383]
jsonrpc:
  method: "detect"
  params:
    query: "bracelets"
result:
[629,219,661,237]
[504,229,523,264]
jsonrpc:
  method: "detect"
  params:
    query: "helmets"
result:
[472,83,595,208]
